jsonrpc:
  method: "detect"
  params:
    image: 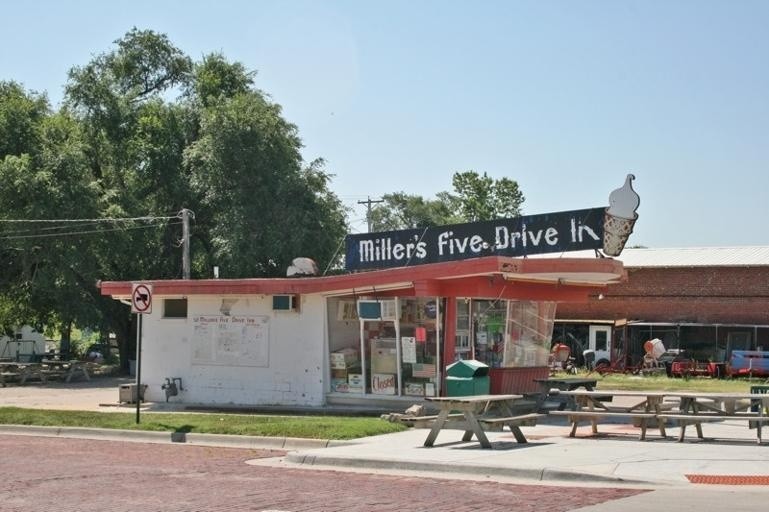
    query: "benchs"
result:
[1,368,93,388]
[402,392,766,448]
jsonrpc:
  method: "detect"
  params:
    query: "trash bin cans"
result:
[444,359,492,396]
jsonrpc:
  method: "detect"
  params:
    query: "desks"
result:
[533,377,599,412]
[41,359,89,382]
[0,359,40,386]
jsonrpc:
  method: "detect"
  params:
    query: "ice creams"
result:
[602,174,640,256]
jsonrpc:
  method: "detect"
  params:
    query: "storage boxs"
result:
[331,348,362,392]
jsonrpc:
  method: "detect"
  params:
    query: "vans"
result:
[730,351,768,370]
